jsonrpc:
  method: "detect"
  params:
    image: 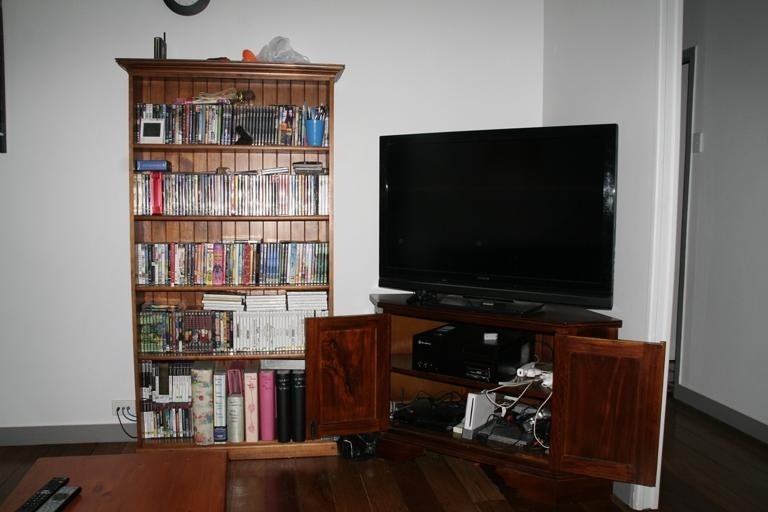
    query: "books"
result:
[141,359,307,446]
[134,160,329,216]
[133,239,330,286]
[139,292,329,353]
[132,86,329,147]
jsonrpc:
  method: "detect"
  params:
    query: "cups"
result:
[305,119,323,146]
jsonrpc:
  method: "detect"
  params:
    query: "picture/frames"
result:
[163,0,210,16]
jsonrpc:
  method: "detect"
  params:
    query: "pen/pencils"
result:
[306,103,326,120]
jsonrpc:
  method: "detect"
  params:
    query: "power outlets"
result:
[112,400,136,416]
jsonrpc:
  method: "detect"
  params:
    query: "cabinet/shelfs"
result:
[114,57,345,462]
[304,295,667,495]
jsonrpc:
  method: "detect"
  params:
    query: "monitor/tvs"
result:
[379,122,618,314]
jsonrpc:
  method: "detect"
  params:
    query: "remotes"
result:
[14,475,69,512]
[35,484,83,512]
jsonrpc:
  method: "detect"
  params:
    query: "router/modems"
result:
[463,392,497,430]
